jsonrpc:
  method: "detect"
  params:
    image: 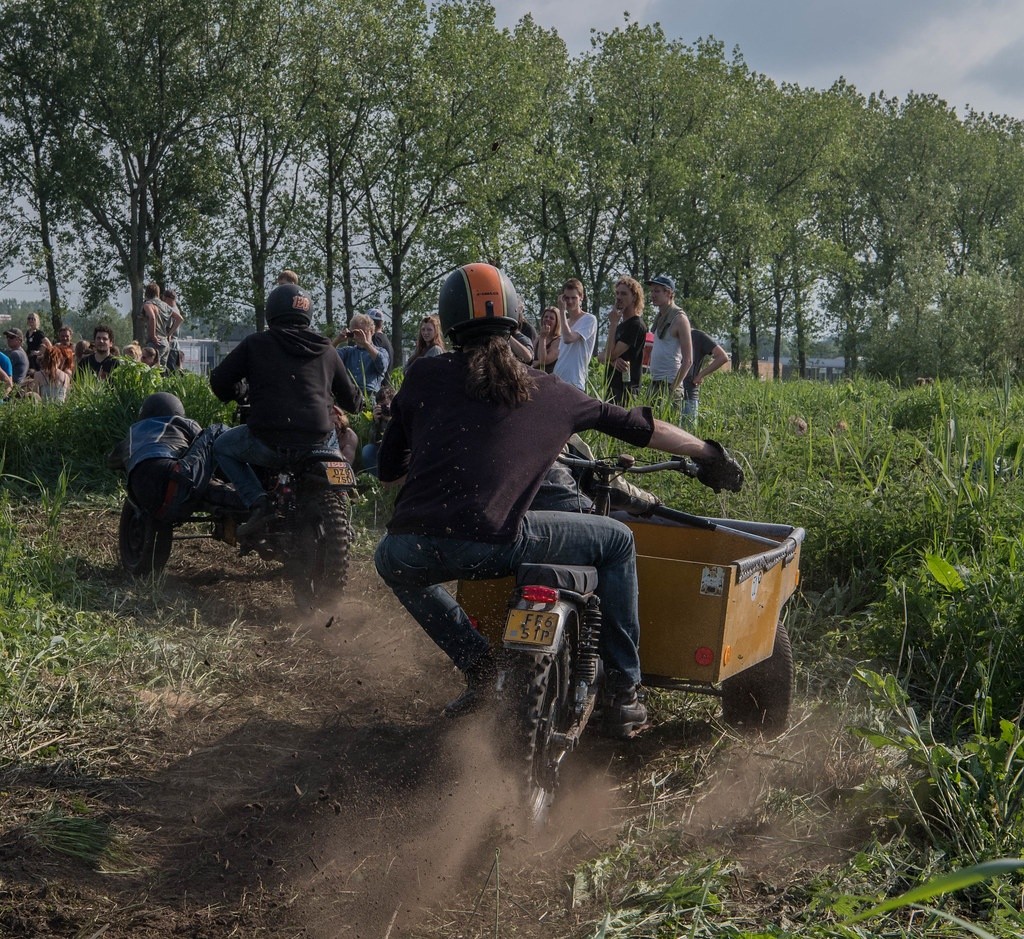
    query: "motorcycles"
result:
[456,443,804,834]
[119,389,359,628]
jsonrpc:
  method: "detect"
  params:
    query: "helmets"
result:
[265,287,314,326]
[439,263,521,338]
[139,393,187,418]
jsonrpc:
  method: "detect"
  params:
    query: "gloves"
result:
[691,438,744,493]
[627,487,662,521]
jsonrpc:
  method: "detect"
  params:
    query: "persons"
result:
[209,285,365,538]
[511,299,538,366]
[142,285,183,374]
[332,387,396,477]
[74,325,120,381]
[680,328,730,428]
[110,341,156,365]
[644,276,693,408]
[278,271,298,286]
[598,276,647,405]
[534,307,562,373]
[0,312,95,406]
[331,309,445,400]
[375,262,745,739]
[119,393,248,521]
[553,279,597,391]
[526,433,660,519]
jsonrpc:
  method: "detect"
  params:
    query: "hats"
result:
[645,277,676,291]
[163,289,177,300]
[367,309,383,321]
[4,328,24,340]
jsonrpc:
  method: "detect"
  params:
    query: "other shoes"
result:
[236,499,285,536]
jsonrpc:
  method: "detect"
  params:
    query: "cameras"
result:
[378,406,390,417]
[346,333,355,337]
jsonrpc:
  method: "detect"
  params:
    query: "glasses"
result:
[6,335,15,338]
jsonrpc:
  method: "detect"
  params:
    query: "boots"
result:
[444,652,506,719]
[603,676,647,735]
